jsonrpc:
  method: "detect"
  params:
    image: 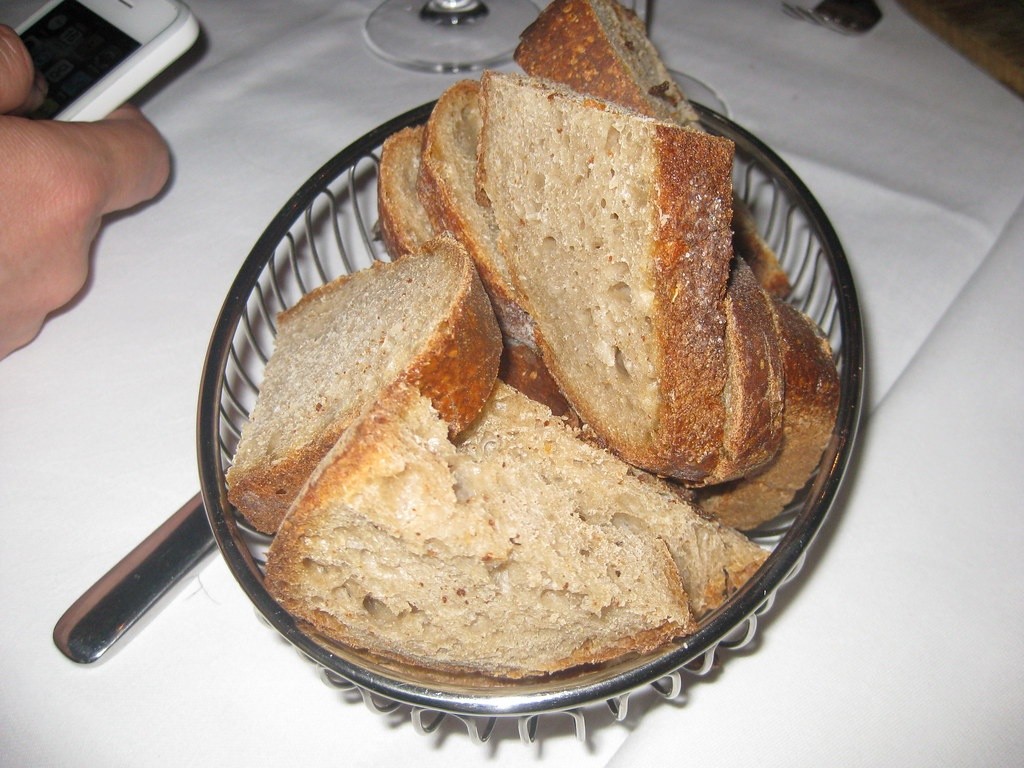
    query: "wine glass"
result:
[363,0,540,74]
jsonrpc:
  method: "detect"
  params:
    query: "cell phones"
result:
[10,0,200,121]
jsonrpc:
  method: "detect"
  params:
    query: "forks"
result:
[780,0,885,37]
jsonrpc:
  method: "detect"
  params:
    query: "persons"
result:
[1,23,172,360]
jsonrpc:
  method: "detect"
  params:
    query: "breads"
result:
[220,0,840,680]
